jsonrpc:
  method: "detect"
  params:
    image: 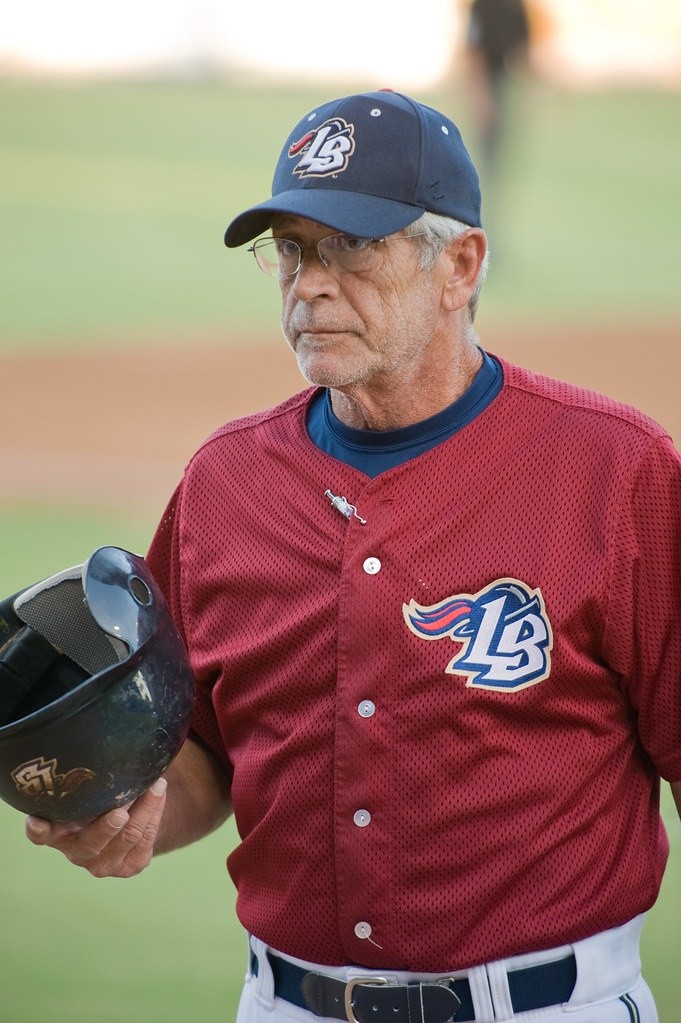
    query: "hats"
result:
[223,87,482,250]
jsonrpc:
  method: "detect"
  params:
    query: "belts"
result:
[251,951,576,1023]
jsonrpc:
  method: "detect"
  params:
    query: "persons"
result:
[465,0,529,166]
[26,87,681,1023]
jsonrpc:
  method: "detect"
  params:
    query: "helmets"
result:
[0,547,197,825]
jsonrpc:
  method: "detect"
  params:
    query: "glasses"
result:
[248,233,433,279]
